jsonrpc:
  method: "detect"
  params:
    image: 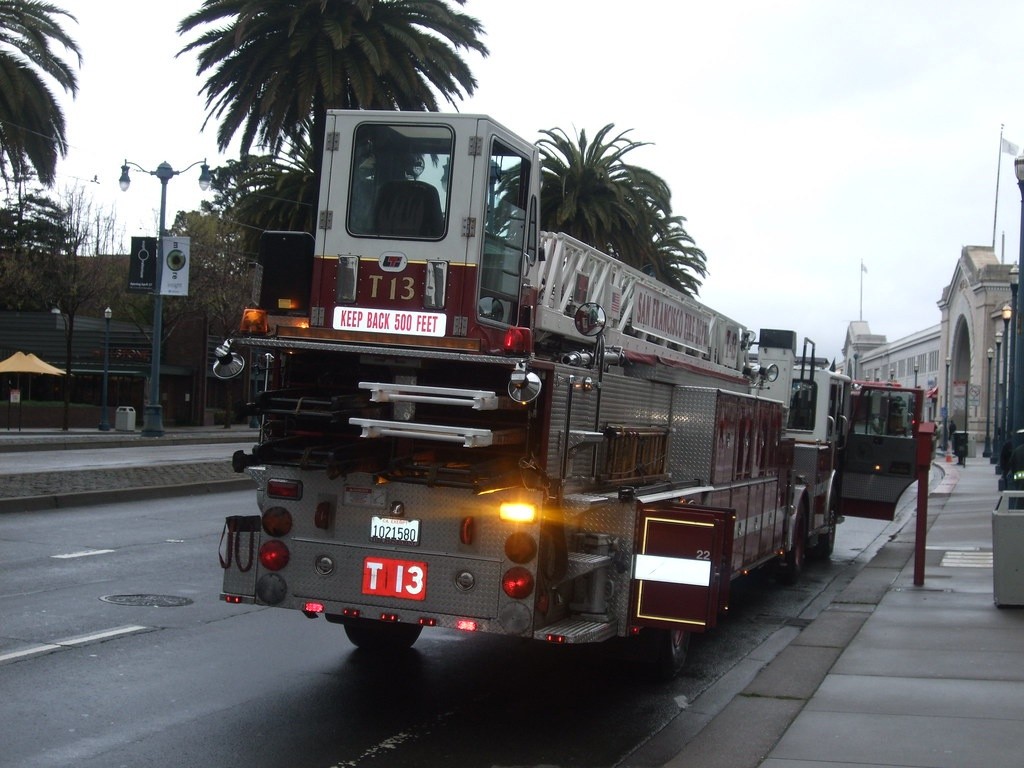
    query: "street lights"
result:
[941,356,953,450]
[889,369,895,380]
[914,364,920,387]
[98,304,113,432]
[118,155,213,437]
[982,262,1019,479]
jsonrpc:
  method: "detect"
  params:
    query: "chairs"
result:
[373,180,444,238]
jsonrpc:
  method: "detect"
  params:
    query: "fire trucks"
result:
[223,107,938,671]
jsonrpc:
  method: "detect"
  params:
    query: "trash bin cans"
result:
[115,406,136,432]
[991,490,1024,607]
